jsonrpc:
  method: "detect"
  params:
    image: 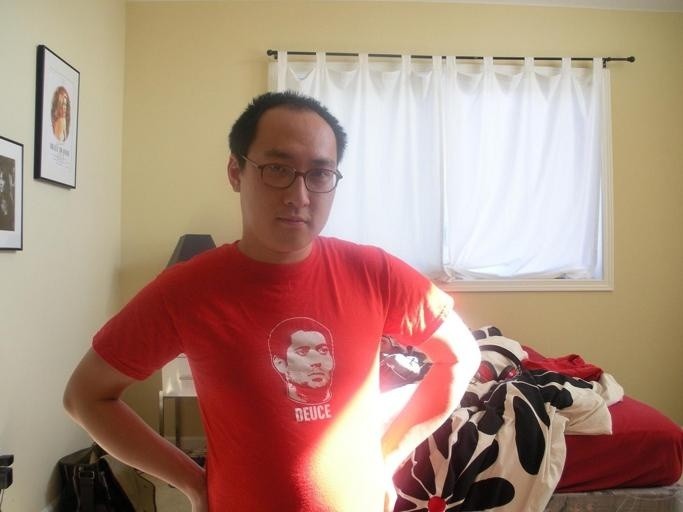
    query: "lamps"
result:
[165,234,217,266]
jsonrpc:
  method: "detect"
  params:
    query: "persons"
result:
[0,166,14,229]
[61,88,483,512]
[268,317,336,406]
[53,92,68,141]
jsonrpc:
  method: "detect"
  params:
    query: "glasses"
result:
[241,155,343,194]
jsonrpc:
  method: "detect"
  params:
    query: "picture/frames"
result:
[32,45,80,190]
[0,135,24,249]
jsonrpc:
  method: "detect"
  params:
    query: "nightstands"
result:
[157,352,199,490]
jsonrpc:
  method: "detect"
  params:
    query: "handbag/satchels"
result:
[57,443,157,512]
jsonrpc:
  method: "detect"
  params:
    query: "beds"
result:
[379,335,682,511]
